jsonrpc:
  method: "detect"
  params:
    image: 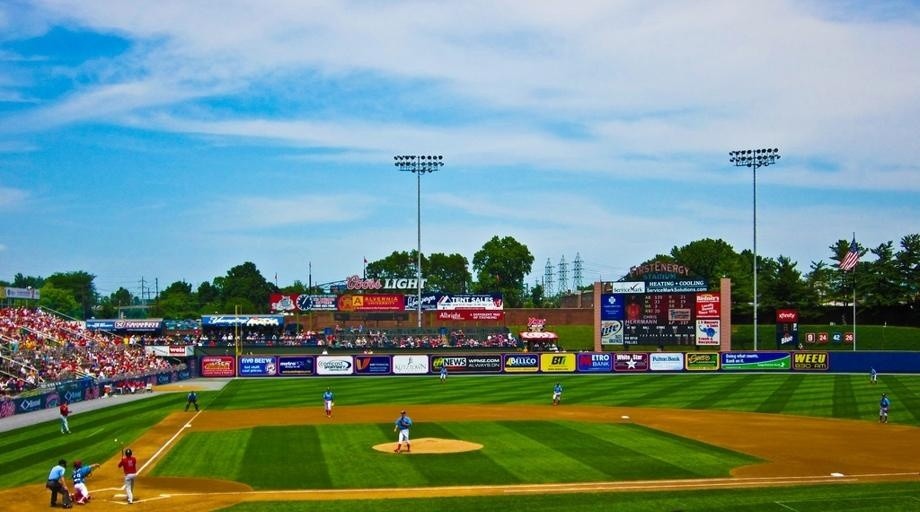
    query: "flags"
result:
[838,238,861,272]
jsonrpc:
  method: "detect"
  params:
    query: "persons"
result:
[394,411,412,452]
[118,449,138,504]
[553,382,562,406]
[125,331,518,355]
[45,459,71,508]
[1,305,178,398]
[439,365,447,384]
[522,340,561,352]
[878,393,892,423]
[70,459,103,506]
[184,391,199,412]
[323,386,334,418]
[869,366,880,386]
[60,399,71,435]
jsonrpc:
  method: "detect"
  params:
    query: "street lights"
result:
[393,153,445,328]
[729,147,781,350]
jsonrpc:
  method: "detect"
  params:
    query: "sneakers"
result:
[185,409,188,412]
[61,432,64,434]
[196,409,199,411]
[77,501,85,504]
[51,503,56,506]
[63,504,72,508]
[84,496,90,501]
[128,502,133,504]
[66,431,71,434]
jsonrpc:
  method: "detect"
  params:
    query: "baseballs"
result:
[115,439,118,441]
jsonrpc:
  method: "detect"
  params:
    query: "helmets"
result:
[125,449,132,455]
[73,460,81,467]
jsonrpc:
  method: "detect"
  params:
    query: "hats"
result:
[400,411,405,414]
[58,460,66,465]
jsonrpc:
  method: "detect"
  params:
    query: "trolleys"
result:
[142,383,153,393]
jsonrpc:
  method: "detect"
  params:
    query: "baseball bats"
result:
[120,441,124,458]
